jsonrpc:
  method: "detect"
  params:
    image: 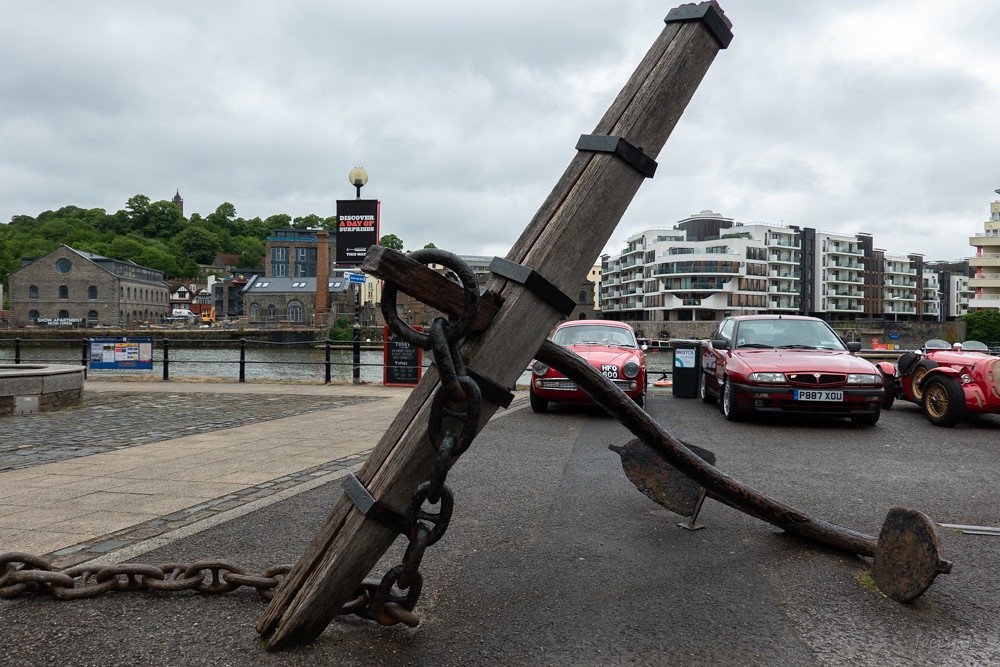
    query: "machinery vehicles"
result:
[201,307,216,325]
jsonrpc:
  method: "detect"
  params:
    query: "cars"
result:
[699,315,886,428]
[530,319,648,414]
[875,338,1000,429]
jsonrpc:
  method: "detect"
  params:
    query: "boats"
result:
[654,380,673,387]
[647,340,673,353]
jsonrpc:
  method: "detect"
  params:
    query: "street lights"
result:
[348,167,369,385]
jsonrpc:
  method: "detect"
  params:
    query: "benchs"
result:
[0,363,87,418]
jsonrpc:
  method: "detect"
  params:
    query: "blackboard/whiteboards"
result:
[383,325,423,387]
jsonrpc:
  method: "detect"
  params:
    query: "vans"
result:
[171,309,195,316]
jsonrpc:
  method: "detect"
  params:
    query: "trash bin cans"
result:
[668,339,706,399]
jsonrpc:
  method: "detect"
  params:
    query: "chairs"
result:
[738,328,757,345]
[792,326,821,346]
